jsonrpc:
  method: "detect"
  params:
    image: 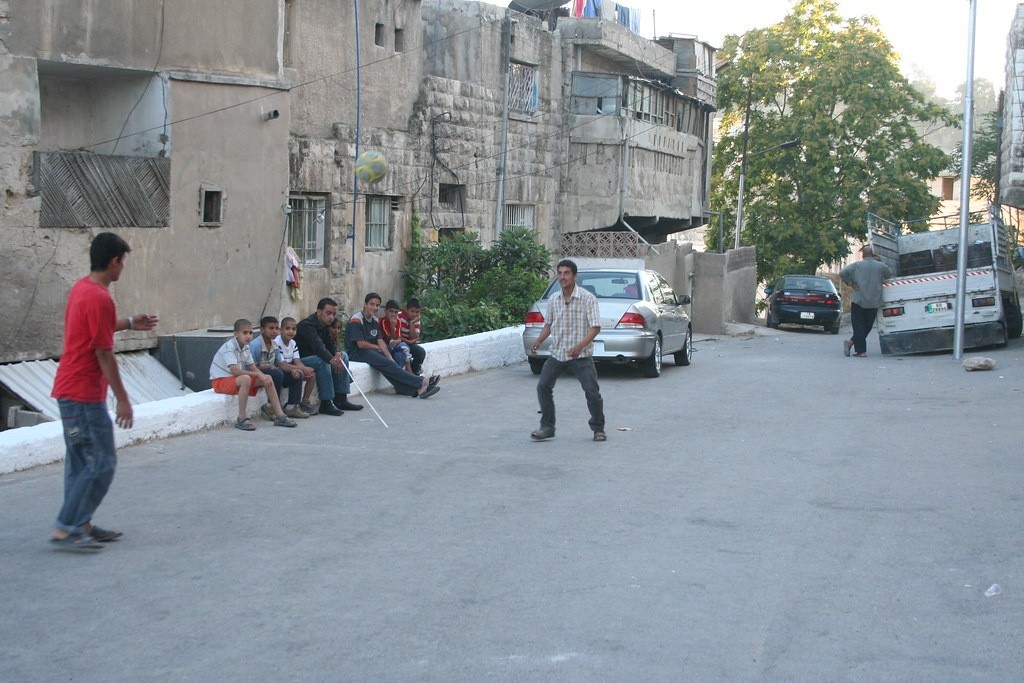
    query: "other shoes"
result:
[333,397,364,410]
[419,385,441,398]
[428,375,440,386]
[531,430,554,439]
[319,399,345,416]
[852,353,867,357]
[843,340,851,357]
[594,431,606,441]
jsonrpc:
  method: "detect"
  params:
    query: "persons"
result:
[271,317,318,415]
[208,318,297,431]
[378,300,413,375]
[43,232,159,554]
[530,259,608,442]
[344,292,440,399]
[839,246,893,357]
[292,298,364,416]
[248,315,310,419]
[398,298,426,377]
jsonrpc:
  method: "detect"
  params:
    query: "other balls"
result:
[355,150,387,184]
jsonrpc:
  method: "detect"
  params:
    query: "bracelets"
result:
[128,316,135,330]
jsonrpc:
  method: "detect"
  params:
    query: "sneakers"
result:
[282,402,310,418]
[260,403,276,420]
[300,400,320,415]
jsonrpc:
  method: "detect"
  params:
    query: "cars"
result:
[763,276,843,335]
[522,269,693,379]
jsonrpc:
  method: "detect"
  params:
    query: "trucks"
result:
[865,220,1024,356]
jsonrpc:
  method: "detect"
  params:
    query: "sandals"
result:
[274,414,298,427]
[235,416,256,430]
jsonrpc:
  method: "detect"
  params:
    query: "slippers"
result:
[87,525,125,542]
[47,531,104,548]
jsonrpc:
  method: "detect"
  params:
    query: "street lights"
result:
[735,138,800,249]
